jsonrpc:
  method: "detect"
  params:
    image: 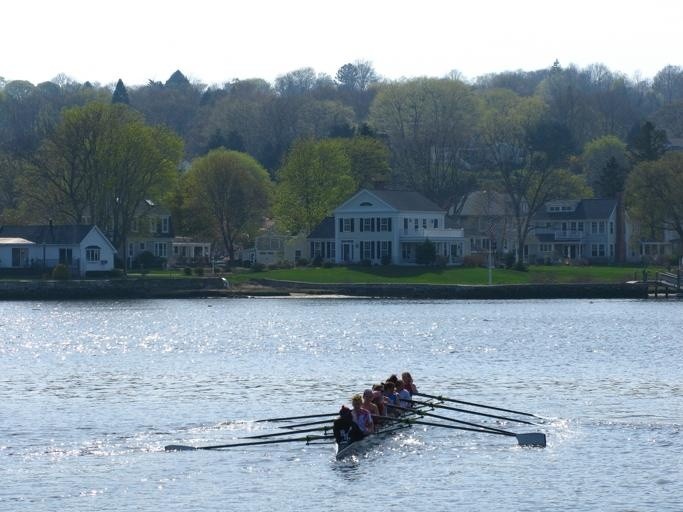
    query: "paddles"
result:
[410,392,558,421]
[398,395,547,427]
[370,403,546,448]
[165,435,336,450]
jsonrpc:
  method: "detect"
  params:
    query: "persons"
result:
[332,372,418,453]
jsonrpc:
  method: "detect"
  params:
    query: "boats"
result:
[334,400,416,461]
